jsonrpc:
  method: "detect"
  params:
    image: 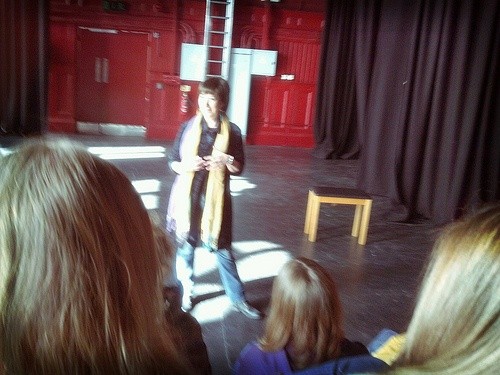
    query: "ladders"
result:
[202,0,234,84]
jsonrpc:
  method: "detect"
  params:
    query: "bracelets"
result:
[226,154,236,167]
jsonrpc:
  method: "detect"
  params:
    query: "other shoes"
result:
[232,300,266,319]
[181,296,193,313]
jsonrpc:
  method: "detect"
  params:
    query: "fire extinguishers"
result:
[178,93,190,122]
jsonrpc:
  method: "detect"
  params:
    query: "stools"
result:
[303,187,373,245]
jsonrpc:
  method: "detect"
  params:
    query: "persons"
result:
[166,74,266,321]
[298,203,500,375]
[0,136,213,374]
[230,257,374,374]
